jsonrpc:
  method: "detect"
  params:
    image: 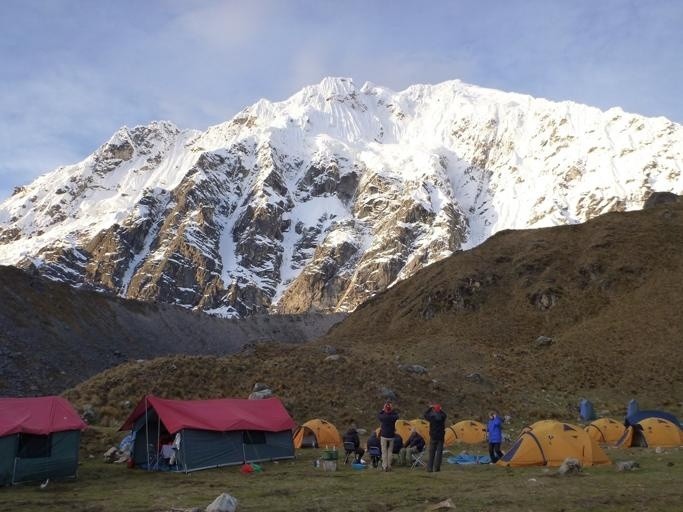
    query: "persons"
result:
[486,410,504,466]
[398,427,425,468]
[366,431,381,469]
[423,403,446,473]
[377,399,398,472]
[343,422,364,465]
[390,428,403,454]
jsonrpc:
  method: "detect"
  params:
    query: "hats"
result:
[384,404,392,414]
[435,404,440,412]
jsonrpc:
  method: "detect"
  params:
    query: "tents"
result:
[115,392,295,475]
[373,420,419,446]
[583,417,625,443]
[611,416,682,450]
[517,420,559,439]
[612,410,681,446]
[292,418,342,450]
[494,421,608,468]
[0,396,88,490]
[410,419,430,447]
[444,420,486,447]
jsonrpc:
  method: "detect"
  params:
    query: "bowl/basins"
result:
[351,463,366,469]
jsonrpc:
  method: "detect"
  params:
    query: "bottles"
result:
[127,456,133,468]
[325,444,336,451]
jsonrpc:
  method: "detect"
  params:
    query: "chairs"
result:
[368,445,385,469]
[343,442,360,465]
[411,445,428,470]
[393,438,403,464]
